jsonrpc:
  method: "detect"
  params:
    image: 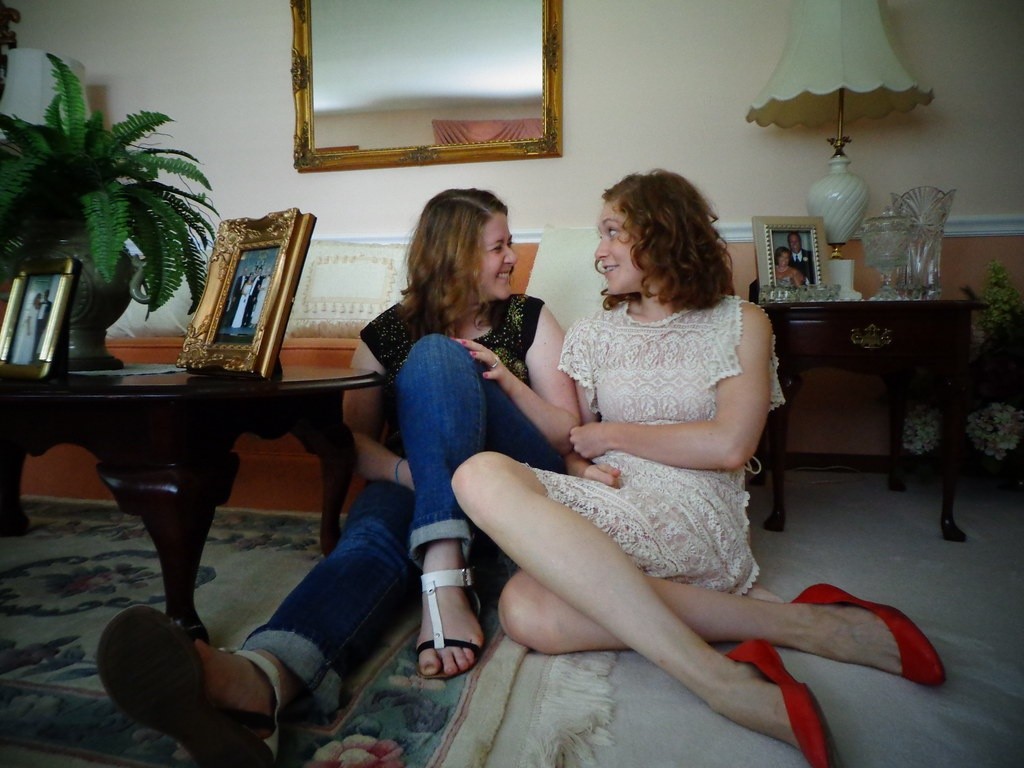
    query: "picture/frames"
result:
[0,254,75,377]
[175,207,316,377]
[751,213,831,299]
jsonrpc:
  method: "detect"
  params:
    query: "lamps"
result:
[744,0,935,301]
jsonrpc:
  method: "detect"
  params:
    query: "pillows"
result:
[103,248,210,339]
[288,241,409,338]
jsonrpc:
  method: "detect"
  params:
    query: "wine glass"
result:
[860,215,913,301]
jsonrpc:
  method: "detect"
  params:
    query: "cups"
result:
[891,186,956,299]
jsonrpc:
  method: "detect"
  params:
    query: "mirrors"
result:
[289,0,564,171]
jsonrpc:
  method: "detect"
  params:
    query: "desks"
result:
[0,373,383,648]
[751,295,987,541]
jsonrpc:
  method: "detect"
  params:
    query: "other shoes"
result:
[790,583,947,686]
[726,639,828,768]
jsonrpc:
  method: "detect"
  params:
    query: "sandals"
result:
[96,605,284,768]
[416,568,483,677]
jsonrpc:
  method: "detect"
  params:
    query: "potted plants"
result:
[0,54,220,374]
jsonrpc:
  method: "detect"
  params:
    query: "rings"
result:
[492,355,501,367]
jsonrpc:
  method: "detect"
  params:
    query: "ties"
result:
[796,255,799,263]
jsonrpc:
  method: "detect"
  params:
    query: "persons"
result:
[217,263,272,328]
[771,231,815,287]
[93,188,582,768]
[448,167,946,768]
[11,290,53,366]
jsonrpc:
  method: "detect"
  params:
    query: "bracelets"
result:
[396,459,405,485]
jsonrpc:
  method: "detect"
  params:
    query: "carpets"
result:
[0,496,616,768]
